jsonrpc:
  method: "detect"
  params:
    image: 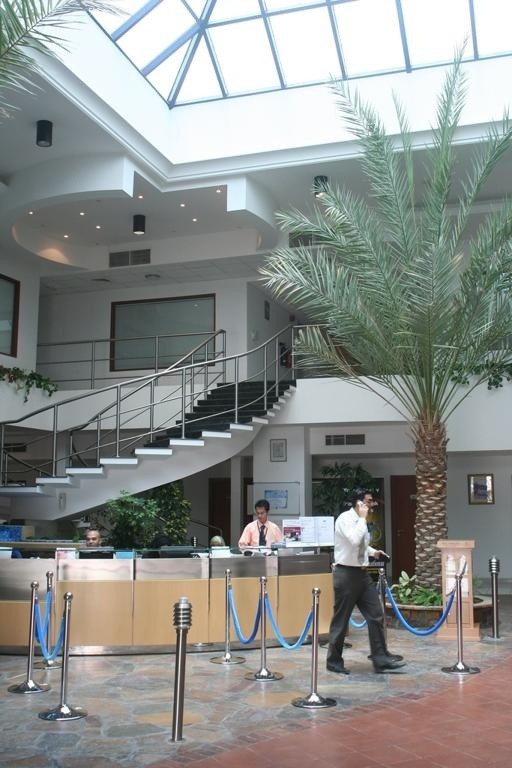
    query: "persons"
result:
[323,491,408,676]
[235,498,284,554]
[74,529,117,561]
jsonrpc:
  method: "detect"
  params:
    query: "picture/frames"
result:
[269,437,287,462]
[468,473,495,504]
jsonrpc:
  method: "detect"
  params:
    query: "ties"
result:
[259,525,266,546]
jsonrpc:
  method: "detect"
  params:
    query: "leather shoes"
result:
[375,662,405,673]
[326,664,350,675]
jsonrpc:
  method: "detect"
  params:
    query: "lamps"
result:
[133,214,145,234]
[313,175,328,197]
[35,120,52,146]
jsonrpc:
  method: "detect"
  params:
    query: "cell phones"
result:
[357,500,364,507]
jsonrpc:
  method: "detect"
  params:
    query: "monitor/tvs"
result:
[79,549,113,559]
[161,546,194,558]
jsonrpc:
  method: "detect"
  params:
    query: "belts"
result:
[336,564,366,569]
